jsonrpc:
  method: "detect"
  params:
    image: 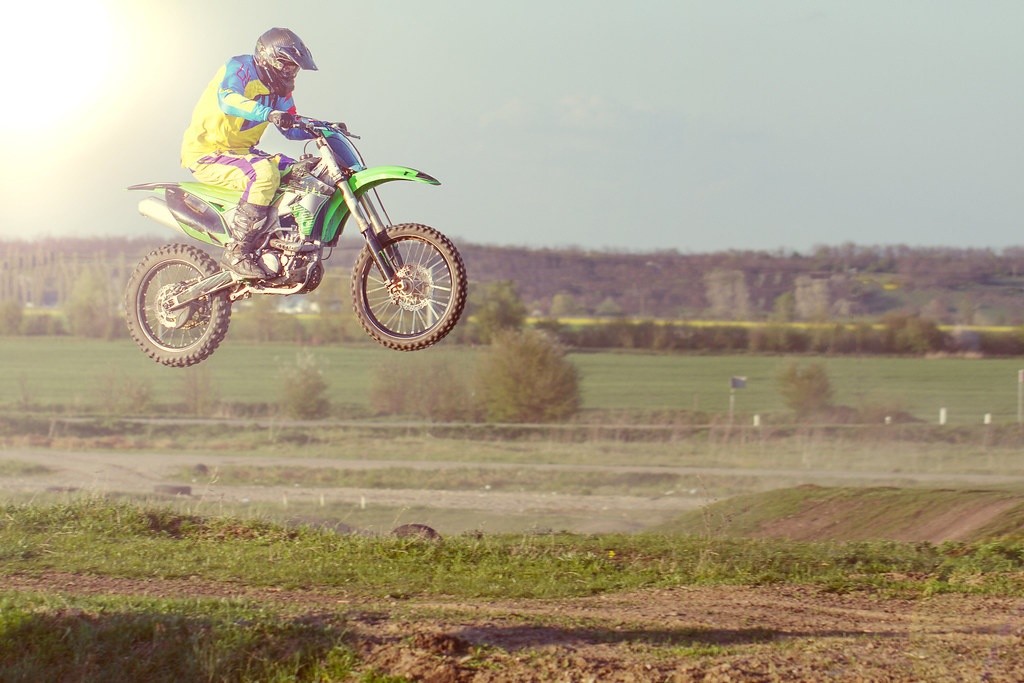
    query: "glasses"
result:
[278,58,300,77]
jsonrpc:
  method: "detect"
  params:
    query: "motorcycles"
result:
[122,114,469,368]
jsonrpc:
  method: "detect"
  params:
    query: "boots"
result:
[219,198,272,280]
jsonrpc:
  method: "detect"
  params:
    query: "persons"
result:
[180,26,350,278]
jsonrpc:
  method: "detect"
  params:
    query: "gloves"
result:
[269,109,294,129]
[330,122,347,132]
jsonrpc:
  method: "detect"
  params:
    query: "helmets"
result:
[253,27,318,93]
